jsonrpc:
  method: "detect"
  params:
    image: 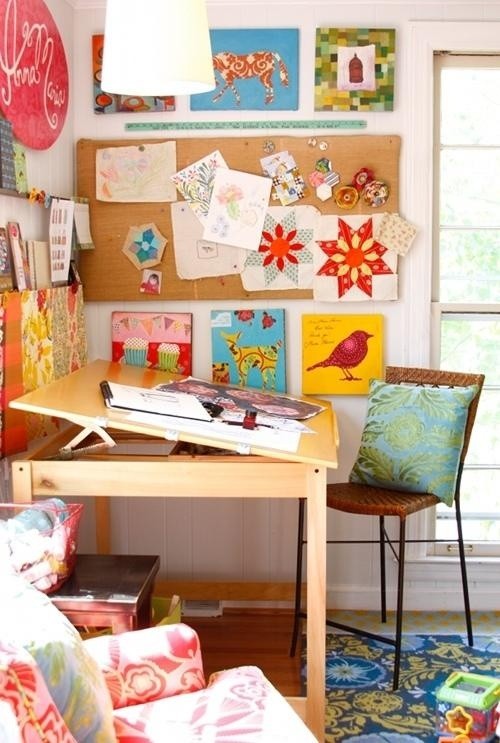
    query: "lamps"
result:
[100,0,216,97]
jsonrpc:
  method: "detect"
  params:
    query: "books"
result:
[100,378,213,423]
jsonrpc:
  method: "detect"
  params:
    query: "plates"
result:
[203,401,224,417]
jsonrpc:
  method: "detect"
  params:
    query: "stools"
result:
[46,554,159,634]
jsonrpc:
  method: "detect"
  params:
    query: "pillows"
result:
[9,576,119,743]
[348,377,480,508]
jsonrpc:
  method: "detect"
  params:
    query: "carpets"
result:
[300,611,500,743]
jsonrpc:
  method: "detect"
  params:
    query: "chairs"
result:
[1,558,319,743]
[291,366,485,690]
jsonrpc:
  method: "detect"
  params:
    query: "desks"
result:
[9,359,337,743]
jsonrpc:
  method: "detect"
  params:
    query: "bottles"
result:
[349,53,363,84]
[243,409,257,430]
